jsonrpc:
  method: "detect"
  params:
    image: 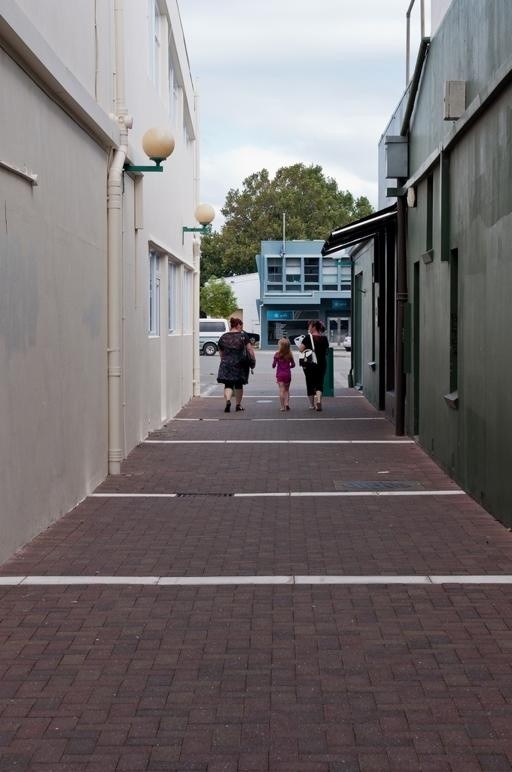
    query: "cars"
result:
[242,330,260,345]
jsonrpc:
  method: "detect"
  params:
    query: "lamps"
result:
[183,205,215,232]
[123,126,176,172]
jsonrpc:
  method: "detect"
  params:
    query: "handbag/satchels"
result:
[240,332,256,369]
[300,334,317,368]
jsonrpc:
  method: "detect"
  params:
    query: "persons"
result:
[272,337,297,411]
[298,319,330,411]
[217,317,257,413]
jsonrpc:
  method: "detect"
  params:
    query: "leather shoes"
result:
[224,400,231,412]
[315,401,321,411]
[236,404,244,411]
[309,405,315,410]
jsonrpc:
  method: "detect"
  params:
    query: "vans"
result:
[199,319,230,356]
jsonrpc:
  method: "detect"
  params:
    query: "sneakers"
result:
[280,408,285,411]
[286,406,289,410]
